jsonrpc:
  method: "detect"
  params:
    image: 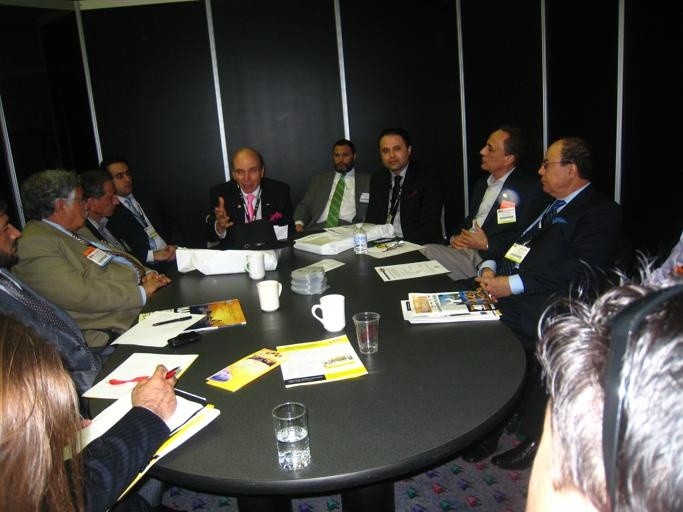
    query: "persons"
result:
[79,158,178,266]
[523,251,682,512]
[287,140,373,235]
[13,170,171,357]
[2,318,178,512]
[364,127,452,247]
[201,144,297,249]
[417,123,541,284]
[2,203,103,393]
[474,134,636,471]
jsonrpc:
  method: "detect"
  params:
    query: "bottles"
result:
[353,223,367,255]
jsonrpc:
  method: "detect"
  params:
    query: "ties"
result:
[492,196,567,284]
[325,171,348,227]
[389,174,403,225]
[245,193,257,224]
[125,199,159,253]
[71,233,148,287]
[2,271,100,374]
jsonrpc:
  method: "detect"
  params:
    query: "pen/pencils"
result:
[165,366,182,380]
[382,244,395,252]
[152,316,192,325]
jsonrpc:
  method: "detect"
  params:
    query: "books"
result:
[65,299,248,512]
[293,217,396,258]
[398,290,504,325]
[272,334,369,391]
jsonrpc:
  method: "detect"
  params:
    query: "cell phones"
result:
[168,331,200,347]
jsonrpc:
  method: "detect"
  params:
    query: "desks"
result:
[87,223,527,512]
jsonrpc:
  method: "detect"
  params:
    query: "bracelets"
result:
[485,241,491,251]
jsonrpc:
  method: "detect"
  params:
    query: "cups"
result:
[351,311,380,355]
[256,279,283,312]
[310,294,346,333]
[271,402,311,472]
[244,252,265,280]
